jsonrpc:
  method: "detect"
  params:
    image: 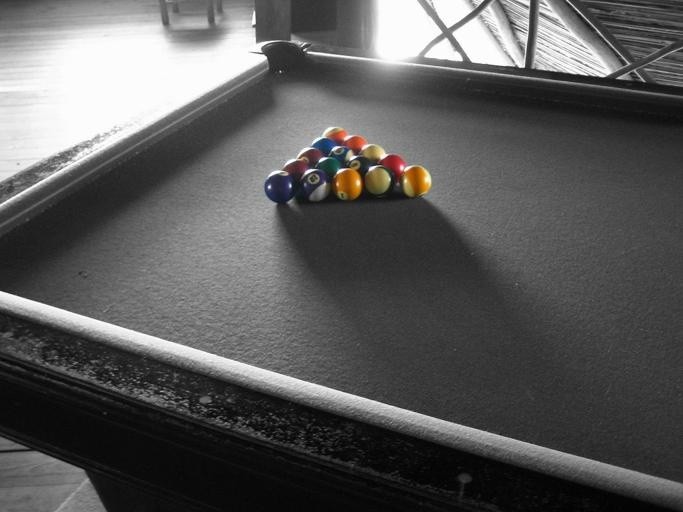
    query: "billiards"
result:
[263,126,431,204]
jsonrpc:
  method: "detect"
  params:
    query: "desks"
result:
[0,36,683,512]
